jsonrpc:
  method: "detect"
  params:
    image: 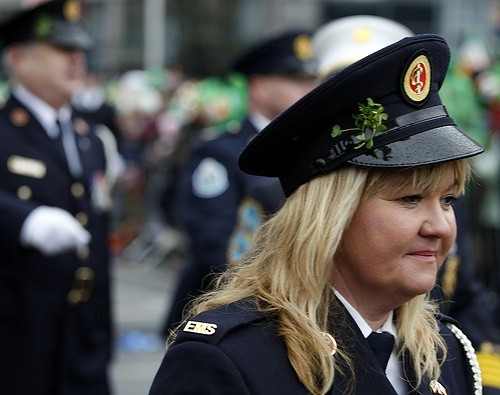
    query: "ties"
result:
[55,120,71,176]
[364,330,395,374]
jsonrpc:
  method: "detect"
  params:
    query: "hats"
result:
[0,0,95,52]
[237,34,484,198]
[231,29,316,81]
[303,15,416,82]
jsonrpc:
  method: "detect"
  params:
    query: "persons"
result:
[439,0,500,288]
[143,15,415,341]
[0,0,126,395]
[147,36,499,395]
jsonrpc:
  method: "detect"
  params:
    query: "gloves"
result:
[21,206,91,256]
